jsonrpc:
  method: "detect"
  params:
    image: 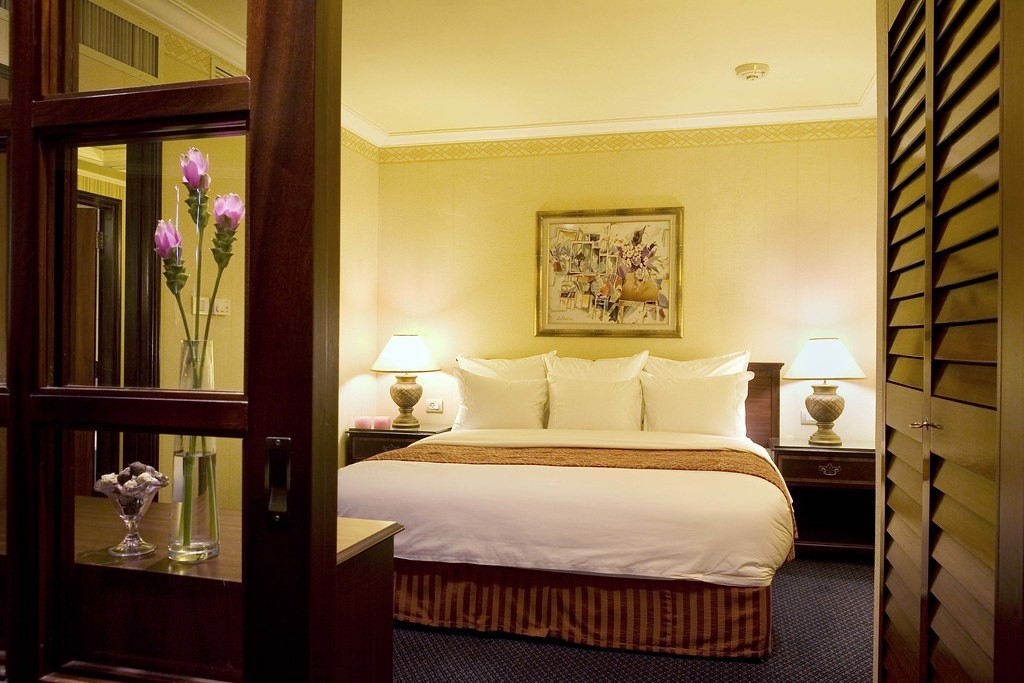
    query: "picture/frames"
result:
[532,202,686,343]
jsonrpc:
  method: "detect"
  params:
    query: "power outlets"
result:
[421,393,444,414]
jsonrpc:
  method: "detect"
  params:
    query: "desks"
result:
[72,496,404,680]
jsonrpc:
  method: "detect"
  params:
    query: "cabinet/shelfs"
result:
[867,0,1024,683]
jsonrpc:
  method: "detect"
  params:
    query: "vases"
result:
[160,334,242,572]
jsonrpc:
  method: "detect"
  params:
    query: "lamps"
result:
[783,331,871,449]
[374,326,443,433]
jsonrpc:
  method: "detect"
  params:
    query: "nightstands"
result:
[346,425,453,465]
[768,426,888,573]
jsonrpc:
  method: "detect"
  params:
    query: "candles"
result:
[353,415,371,432]
[374,413,391,433]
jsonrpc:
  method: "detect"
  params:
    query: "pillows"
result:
[537,350,649,435]
[442,350,554,436]
[636,351,760,443]
[640,371,755,438]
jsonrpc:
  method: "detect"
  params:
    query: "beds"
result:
[330,345,800,663]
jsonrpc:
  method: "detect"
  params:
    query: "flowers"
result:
[148,145,250,331]
